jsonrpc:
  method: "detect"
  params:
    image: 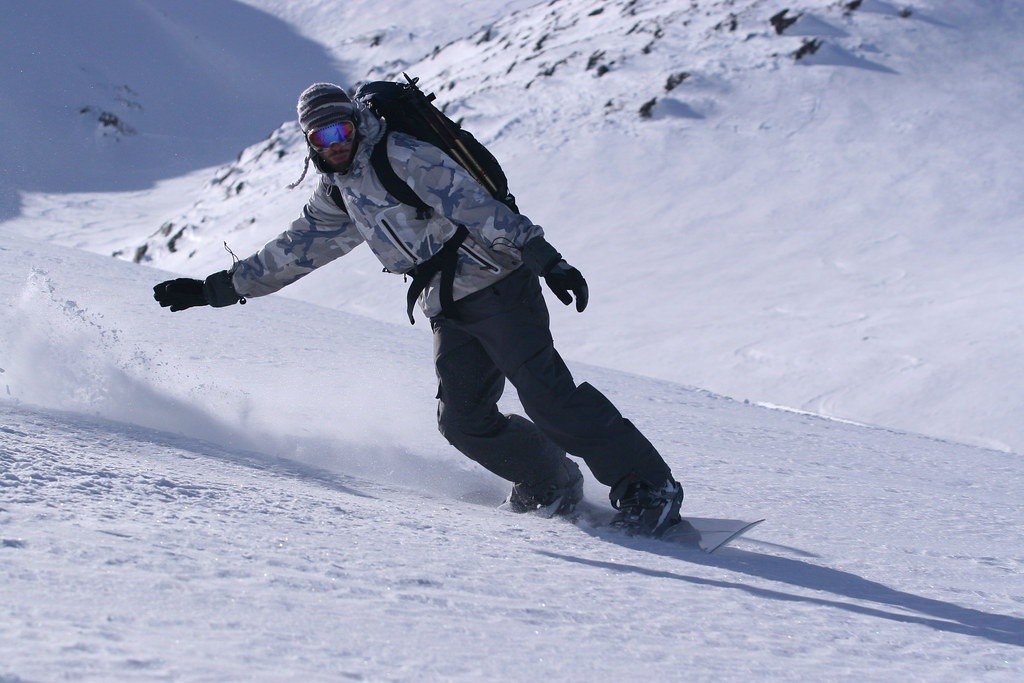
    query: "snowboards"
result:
[503,486,765,554]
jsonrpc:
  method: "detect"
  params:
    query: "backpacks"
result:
[321,72,520,326]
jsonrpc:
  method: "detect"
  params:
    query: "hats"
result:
[284,83,387,193]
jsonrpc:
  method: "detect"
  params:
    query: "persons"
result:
[153,82,683,541]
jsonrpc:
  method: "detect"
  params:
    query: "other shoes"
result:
[607,479,684,540]
[498,458,584,521]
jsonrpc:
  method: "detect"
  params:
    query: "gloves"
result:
[153,240,248,313]
[490,233,589,313]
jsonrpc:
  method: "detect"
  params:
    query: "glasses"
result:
[307,119,357,153]
